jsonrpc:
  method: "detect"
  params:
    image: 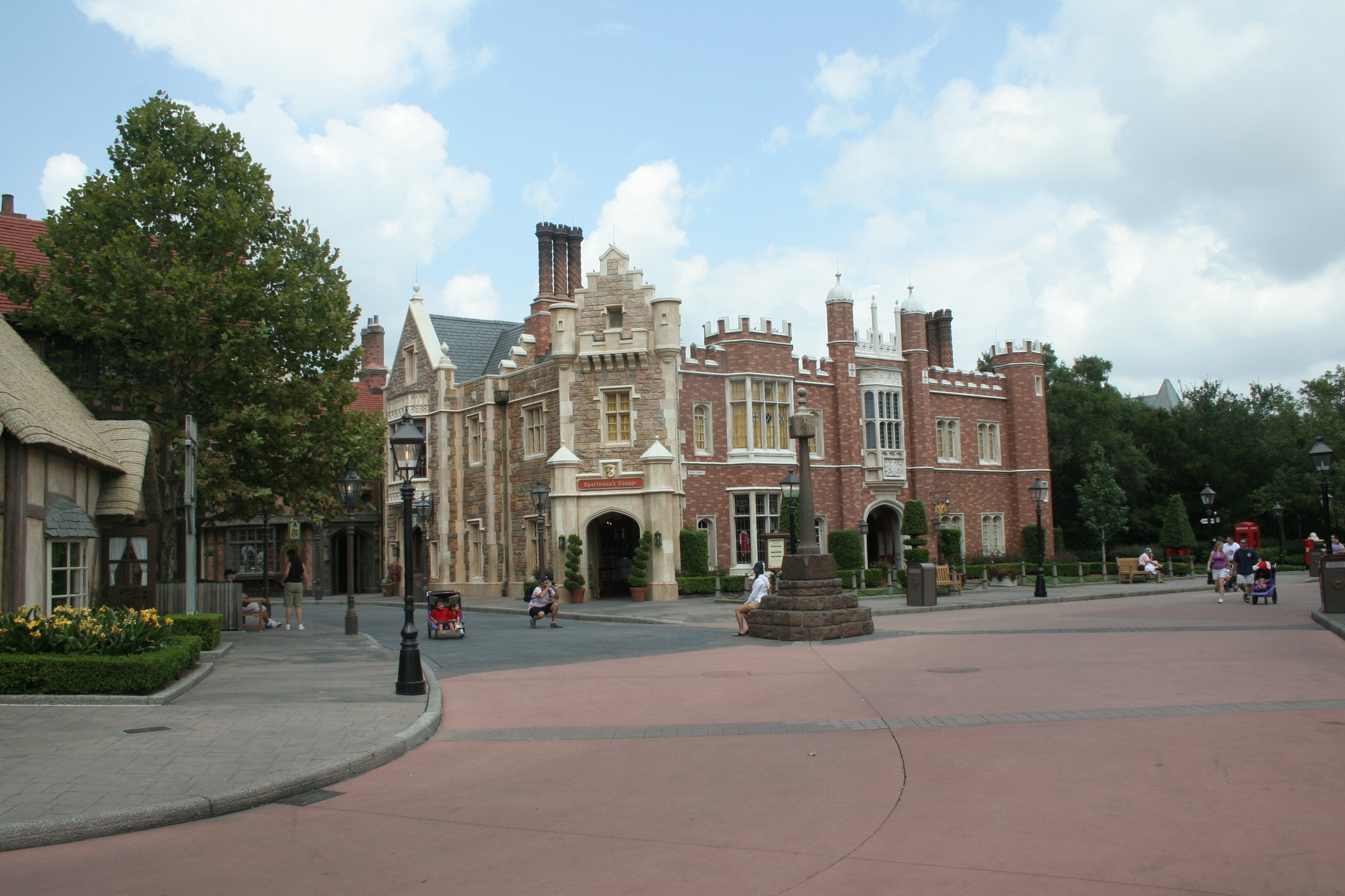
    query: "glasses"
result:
[545,582,550,584]
[1240,542,1246,544]
[1332,537,1335,539]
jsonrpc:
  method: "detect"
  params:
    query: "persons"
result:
[1254,578,1267,590]
[739,530,751,564]
[527,576,562,628]
[1233,538,1262,603]
[1222,535,1240,587]
[449,601,465,637]
[1139,548,1165,583]
[224,569,283,628]
[1224,565,1233,584]
[1207,540,1233,604]
[280,549,306,630]
[429,597,458,636]
[1322,534,1345,554]
[732,562,770,636]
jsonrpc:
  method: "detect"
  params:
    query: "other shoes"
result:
[1218,598,1224,603]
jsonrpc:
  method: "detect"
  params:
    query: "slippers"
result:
[746,630,749,635]
[1155,566,1162,570]
[732,632,746,636]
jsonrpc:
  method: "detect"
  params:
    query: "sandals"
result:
[1157,580,1165,583]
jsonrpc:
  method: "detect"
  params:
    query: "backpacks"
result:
[524,585,543,603]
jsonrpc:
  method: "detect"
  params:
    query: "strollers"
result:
[1250,561,1278,605]
[426,589,466,640]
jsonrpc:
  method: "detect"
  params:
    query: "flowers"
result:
[988,565,1021,582]
[280,542,298,552]
[381,578,393,585]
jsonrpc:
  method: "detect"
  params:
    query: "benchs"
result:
[904,562,964,595]
[1115,557,1152,585]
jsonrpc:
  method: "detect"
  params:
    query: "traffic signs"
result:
[1200,518,1215,524]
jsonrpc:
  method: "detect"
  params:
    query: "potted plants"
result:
[627,530,654,601]
[871,557,893,586]
[388,562,402,596]
[563,533,586,603]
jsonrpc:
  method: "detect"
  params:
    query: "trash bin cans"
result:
[1309,548,1327,577]
[1318,552,1345,613]
[906,562,937,606]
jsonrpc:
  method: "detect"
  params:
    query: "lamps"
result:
[392,539,400,560]
[655,530,663,547]
[856,519,869,535]
[558,534,566,550]
[931,518,942,532]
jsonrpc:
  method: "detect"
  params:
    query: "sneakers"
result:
[298,624,305,630]
[530,619,536,628]
[1246,597,1251,603]
[460,632,465,636]
[265,623,274,628]
[286,624,290,630]
[274,622,282,627]
[1243,593,1248,601]
[550,622,562,628]
[455,625,464,630]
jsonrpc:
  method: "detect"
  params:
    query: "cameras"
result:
[550,581,555,585]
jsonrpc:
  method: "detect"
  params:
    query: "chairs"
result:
[243,598,266,632]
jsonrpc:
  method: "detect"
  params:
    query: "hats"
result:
[224,569,238,576]
[539,575,548,581]
[1145,547,1152,553]
[752,562,764,572]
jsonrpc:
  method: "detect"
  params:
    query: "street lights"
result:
[1028,477,1048,597]
[334,454,359,635]
[1271,501,1286,565]
[390,403,428,696]
[1309,435,1334,553]
[529,476,550,581]
[778,467,800,555]
[1198,480,1217,584]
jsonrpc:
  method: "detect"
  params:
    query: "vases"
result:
[382,584,396,598]
[990,576,1017,586]
